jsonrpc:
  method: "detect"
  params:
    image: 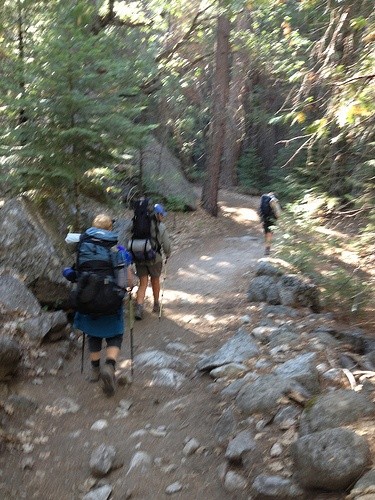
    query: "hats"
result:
[153,204,168,217]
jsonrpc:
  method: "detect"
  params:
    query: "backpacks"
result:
[129,198,161,262]
[70,227,132,318]
[259,196,275,218]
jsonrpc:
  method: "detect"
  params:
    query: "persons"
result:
[133,204,171,321]
[74,214,132,397]
[257,192,280,255]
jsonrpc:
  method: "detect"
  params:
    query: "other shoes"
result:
[152,302,165,312]
[265,246,270,255]
[101,370,115,396]
[92,368,103,382]
[133,302,144,320]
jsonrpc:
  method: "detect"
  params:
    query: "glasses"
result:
[108,219,115,228]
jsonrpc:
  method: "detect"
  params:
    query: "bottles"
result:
[63,267,75,280]
